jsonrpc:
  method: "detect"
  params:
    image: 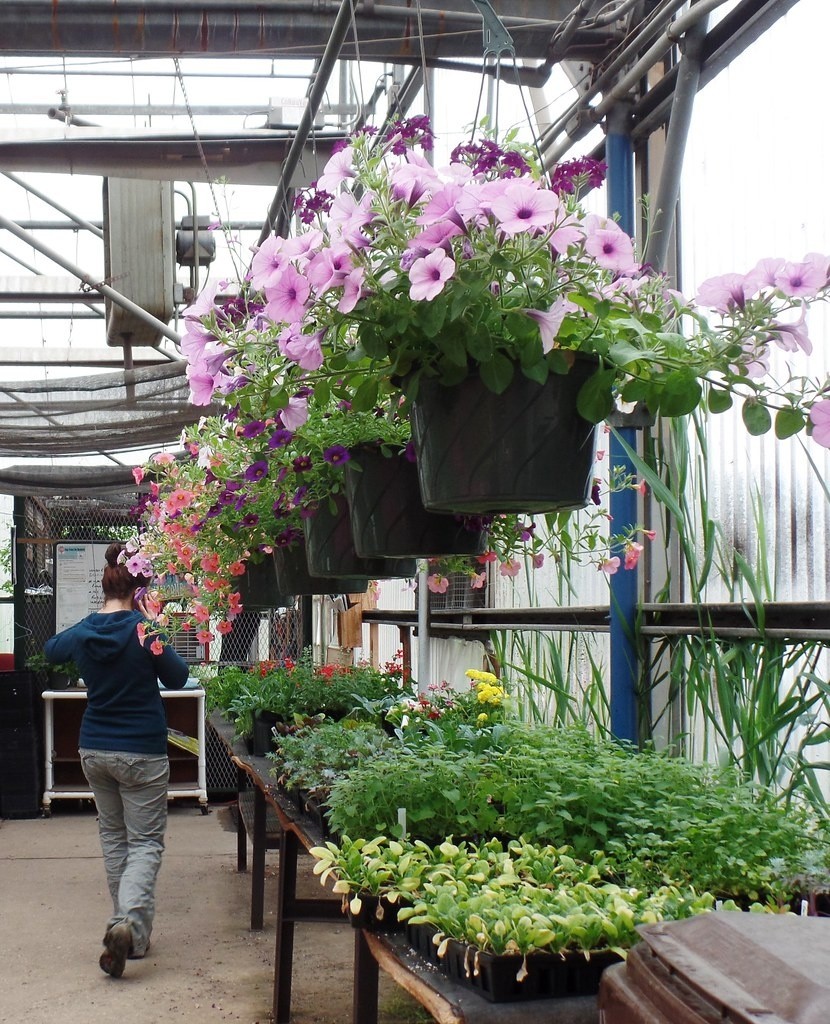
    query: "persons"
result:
[47,544,188,986]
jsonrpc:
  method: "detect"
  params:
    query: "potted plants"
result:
[24,650,80,690]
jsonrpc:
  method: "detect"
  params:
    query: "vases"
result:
[410,351,596,517]
[229,557,293,609]
[273,544,369,595]
[343,443,486,559]
[302,496,415,581]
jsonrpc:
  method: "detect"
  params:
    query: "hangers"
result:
[0,612,32,643]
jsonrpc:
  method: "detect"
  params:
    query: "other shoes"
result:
[99,924,131,979]
[130,941,152,958]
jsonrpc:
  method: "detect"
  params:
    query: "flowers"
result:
[116,114,830,727]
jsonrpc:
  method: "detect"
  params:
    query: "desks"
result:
[41,683,597,1024]
